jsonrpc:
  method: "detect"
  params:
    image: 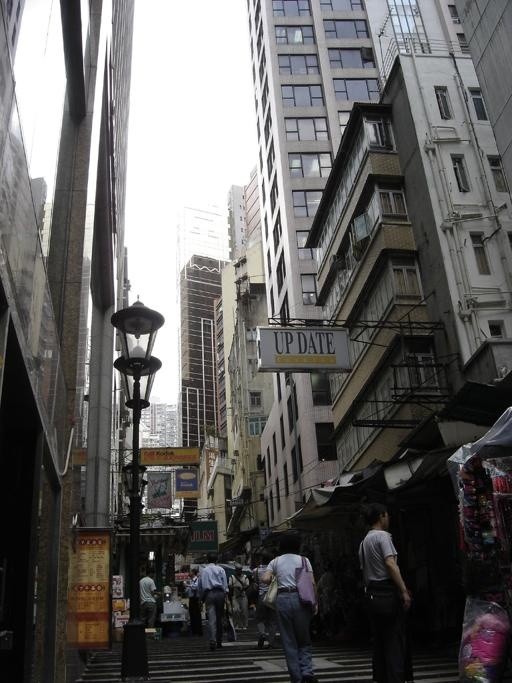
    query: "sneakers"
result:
[211,639,221,649]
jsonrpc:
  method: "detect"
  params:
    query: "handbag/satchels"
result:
[295,567,316,607]
[262,574,277,609]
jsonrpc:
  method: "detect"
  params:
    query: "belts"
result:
[278,589,295,592]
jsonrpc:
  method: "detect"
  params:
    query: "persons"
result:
[362,501,412,682]
[358,520,415,682]
[180,567,206,637]
[246,543,416,650]
[257,532,322,681]
[228,561,249,631]
[197,549,229,648]
[137,567,164,629]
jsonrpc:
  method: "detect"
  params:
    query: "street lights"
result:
[109,294,164,681]
